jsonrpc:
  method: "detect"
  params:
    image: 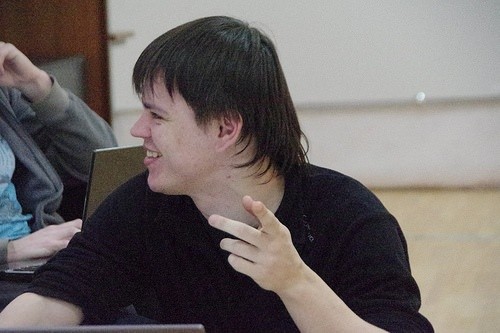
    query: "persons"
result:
[0,40,163,325]
[0,15,435,333]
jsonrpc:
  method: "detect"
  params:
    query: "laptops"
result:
[0,146,147,283]
